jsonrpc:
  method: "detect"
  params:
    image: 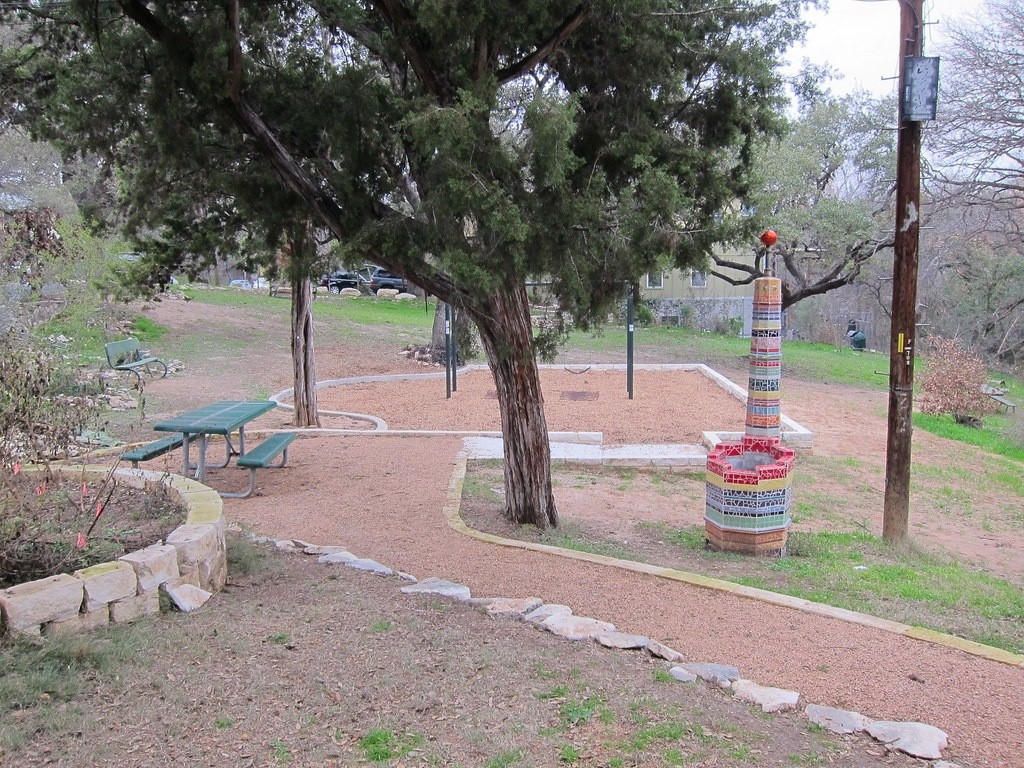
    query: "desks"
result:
[153,400,278,497]
[979,384,1002,398]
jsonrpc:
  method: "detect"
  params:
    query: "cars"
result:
[229,279,251,288]
[112,252,147,275]
[320,265,378,294]
[253,278,270,289]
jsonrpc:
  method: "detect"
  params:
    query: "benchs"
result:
[120,433,201,473]
[237,431,299,498]
[990,396,1017,413]
[104,337,169,389]
[269,282,317,301]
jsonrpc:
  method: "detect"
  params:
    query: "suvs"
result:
[370,267,407,296]
